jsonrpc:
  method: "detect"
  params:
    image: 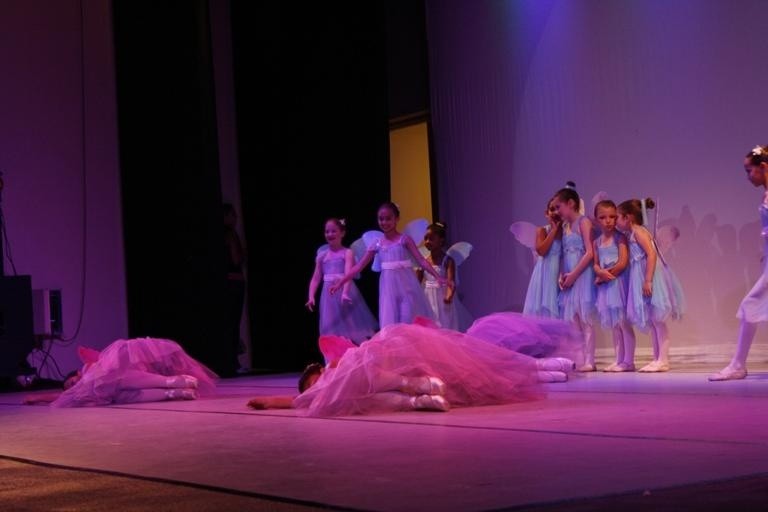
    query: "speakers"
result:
[34,288,64,338]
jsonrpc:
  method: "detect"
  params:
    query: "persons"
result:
[221,201,248,380]
[554,180,597,374]
[461,312,583,385]
[706,145,768,382]
[616,197,687,372]
[20,338,218,410]
[244,316,548,414]
[328,201,455,331]
[306,217,378,366]
[509,197,563,320]
[591,199,636,373]
[415,217,474,331]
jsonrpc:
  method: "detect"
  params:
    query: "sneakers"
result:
[164,389,197,400]
[167,375,199,390]
[537,358,576,372]
[547,371,569,383]
[400,375,446,395]
[638,364,652,373]
[612,361,635,372]
[409,395,450,412]
[578,364,596,372]
[644,362,669,373]
[603,363,616,372]
[708,364,748,382]
